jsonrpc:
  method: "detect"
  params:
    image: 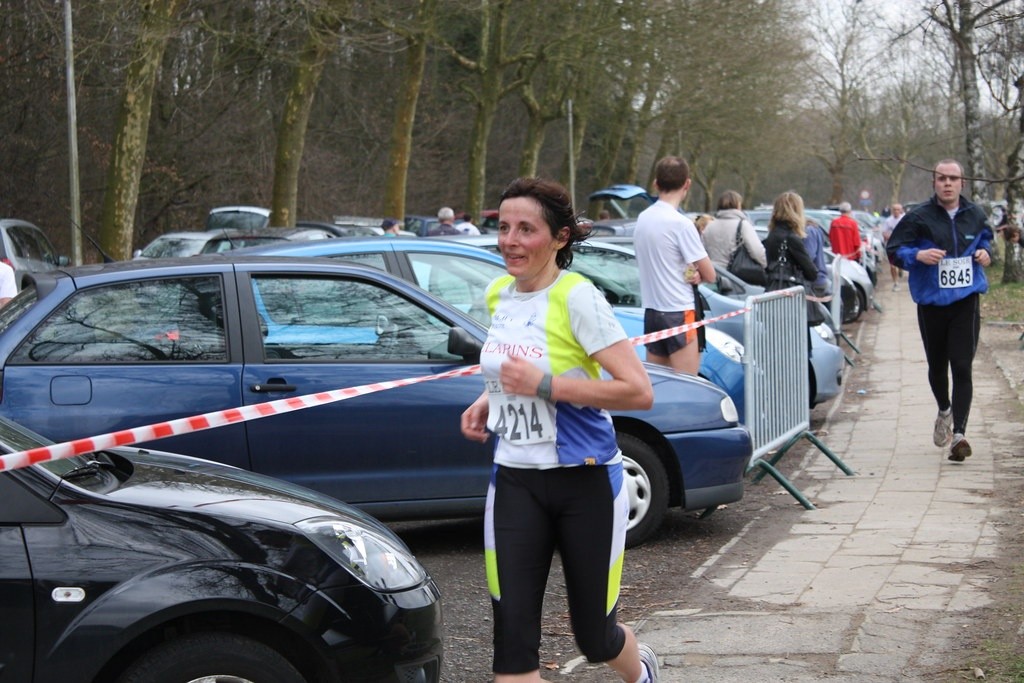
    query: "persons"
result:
[887,159,994,462]
[801,216,826,297]
[700,191,767,270]
[426,206,461,237]
[600,210,609,220]
[378,218,404,236]
[459,177,658,683]
[994,205,1024,249]
[632,156,717,377]
[829,202,862,264]
[0,262,17,308]
[873,203,906,291]
[456,213,481,235]
[763,192,825,409]
[694,214,716,235]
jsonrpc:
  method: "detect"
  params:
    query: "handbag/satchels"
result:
[764,232,825,327]
[727,218,766,286]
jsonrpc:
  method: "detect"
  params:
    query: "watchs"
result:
[534,372,553,400]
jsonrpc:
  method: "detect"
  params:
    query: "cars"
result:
[145,234,766,427]
[0,254,755,551]
[0,413,446,683]
[0,184,923,416]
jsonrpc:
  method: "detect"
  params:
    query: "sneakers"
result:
[933,404,953,447]
[948,434,972,462]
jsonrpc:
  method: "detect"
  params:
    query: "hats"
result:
[382,217,400,230]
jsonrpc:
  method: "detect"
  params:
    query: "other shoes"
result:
[638,643,660,683]
[892,283,900,292]
[898,268,906,278]
